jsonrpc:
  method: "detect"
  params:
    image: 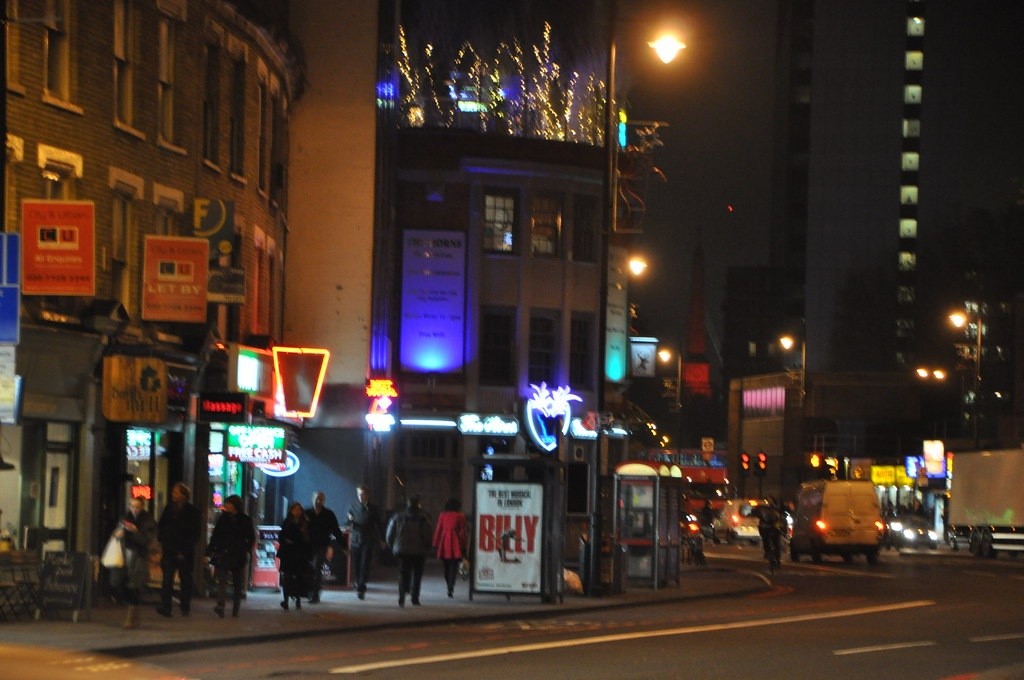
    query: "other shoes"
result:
[157,604,173,618]
[309,595,320,604]
[124,604,140,629]
[214,606,225,617]
[398,598,405,607]
[411,598,420,607]
[281,602,289,610]
[359,591,365,600]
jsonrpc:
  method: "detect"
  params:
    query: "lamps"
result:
[0,458,15,470]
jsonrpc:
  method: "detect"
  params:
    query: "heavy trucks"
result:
[940,450,1024,558]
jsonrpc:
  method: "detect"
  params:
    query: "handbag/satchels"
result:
[459,559,470,581]
[102,536,124,567]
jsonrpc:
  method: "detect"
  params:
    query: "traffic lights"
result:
[758,453,766,472]
[741,453,749,476]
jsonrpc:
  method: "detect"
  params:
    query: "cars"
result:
[946,529,969,549]
[884,516,937,550]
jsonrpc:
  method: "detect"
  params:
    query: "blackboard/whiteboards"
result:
[34,551,92,609]
[319,528,351,591]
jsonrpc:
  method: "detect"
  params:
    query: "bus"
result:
[648,448,730,537]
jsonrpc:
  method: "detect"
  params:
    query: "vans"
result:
[713,498,770,544]
[789,480,886,564]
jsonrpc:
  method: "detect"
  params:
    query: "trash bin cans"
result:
[579,532,617,593]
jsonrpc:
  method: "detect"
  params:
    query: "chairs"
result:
[0,552,60,621]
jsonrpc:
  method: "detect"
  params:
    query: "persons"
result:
[764,524,782,568]
[112,482,470,627]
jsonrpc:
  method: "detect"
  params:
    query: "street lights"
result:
[657,344,684,453]
[589,29,687,591]
[948,309,981,448]
[778,334,807,482]
[917,368,945,440]
